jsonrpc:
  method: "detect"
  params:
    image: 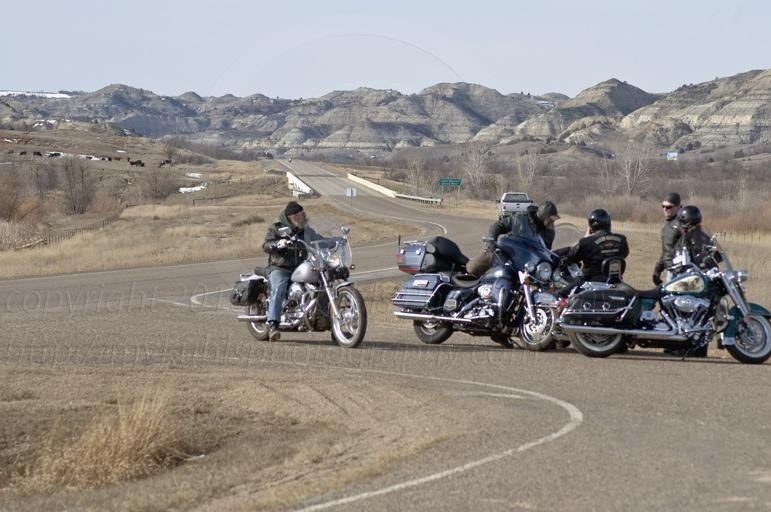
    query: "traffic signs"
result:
[439,178,462,186]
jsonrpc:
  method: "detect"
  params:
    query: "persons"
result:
[262,202,347,342]
[565,209,628,293]
[653,193,695,356]
[664,205,724,357]
[465,201,561,275]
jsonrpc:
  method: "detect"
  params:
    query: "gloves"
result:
[652,261,663,285]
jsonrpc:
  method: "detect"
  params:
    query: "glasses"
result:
[661,205,672,209]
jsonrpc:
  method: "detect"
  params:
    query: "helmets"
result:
[536,201,561,222]
[587,208,611,232]
[677,206,702,224]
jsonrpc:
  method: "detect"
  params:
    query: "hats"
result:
[284,201,304,216]
[663,192,681,206]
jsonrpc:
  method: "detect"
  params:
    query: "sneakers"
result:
[268,326,280,342]
[491,336,513,349]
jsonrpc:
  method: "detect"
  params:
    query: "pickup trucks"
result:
[496,191,533,220]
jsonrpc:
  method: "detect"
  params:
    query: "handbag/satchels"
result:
[229,278,264,306]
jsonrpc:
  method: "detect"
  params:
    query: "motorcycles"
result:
[559,234,771,363]
[551,242,626,348]
[393,215,562,351]
[230,226,368,348]
